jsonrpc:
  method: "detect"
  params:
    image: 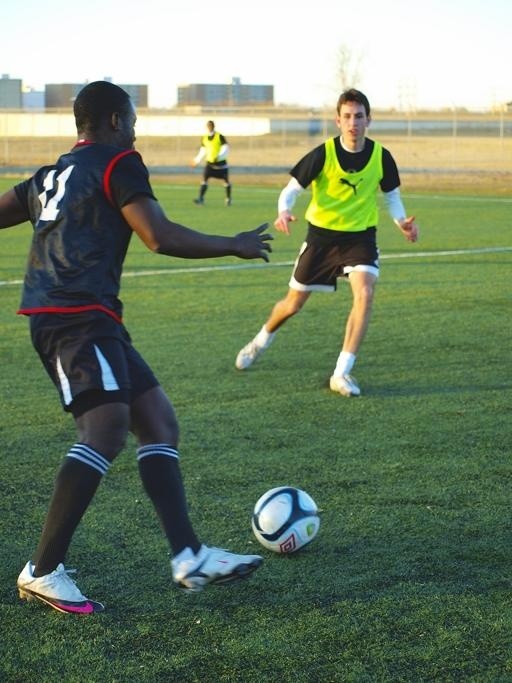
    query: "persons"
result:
[2,77,279,618]
[188,120,236,206]
[234,85,419,397]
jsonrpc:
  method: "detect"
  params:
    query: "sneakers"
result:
[235,341,266,370]
[193,198,203,205]
[170,544,264,593]
[226,199,231,205]
[16,561,105,615]
[330,375,361,397]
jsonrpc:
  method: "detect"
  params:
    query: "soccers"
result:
[254,485,320,553]
